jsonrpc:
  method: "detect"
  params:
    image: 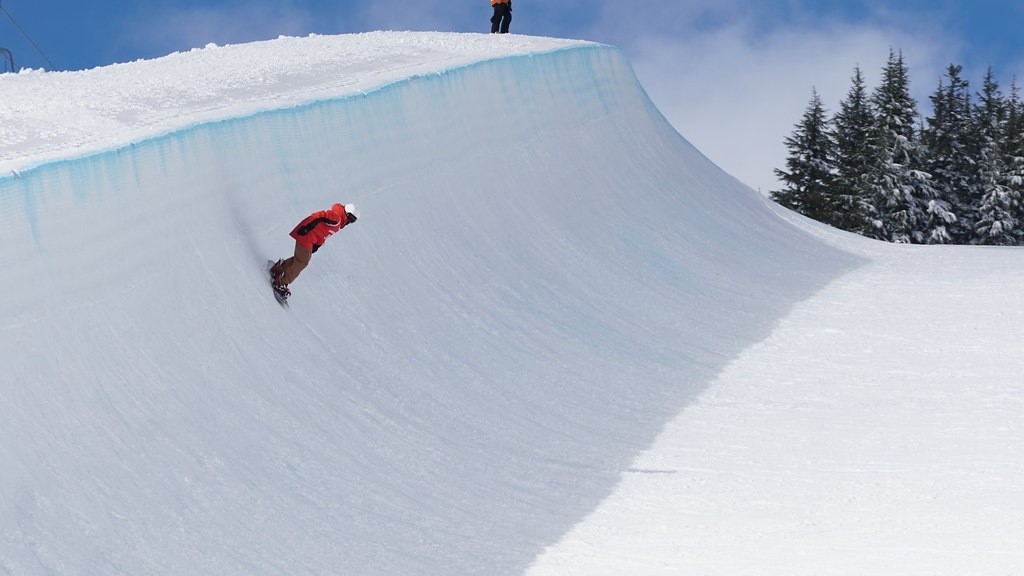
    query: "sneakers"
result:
[270,258,285,275]
[270,274,291,298]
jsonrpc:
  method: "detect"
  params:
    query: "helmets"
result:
[345,204,360,219]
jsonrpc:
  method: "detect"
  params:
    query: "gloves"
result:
[312,244,321,253]
[296,225,307,236]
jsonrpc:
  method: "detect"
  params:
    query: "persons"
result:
[490,0,512,34]
[269,203,360,305]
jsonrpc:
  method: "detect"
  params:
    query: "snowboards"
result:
[273,257,290,310]
